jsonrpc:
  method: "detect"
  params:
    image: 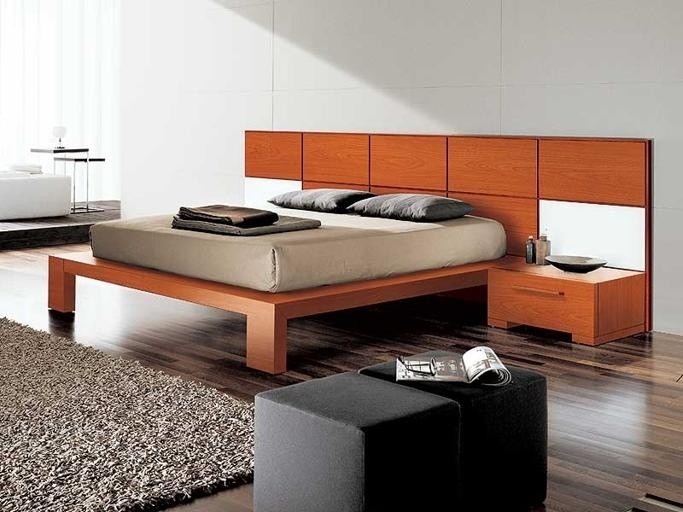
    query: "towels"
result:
[178,203,278,228]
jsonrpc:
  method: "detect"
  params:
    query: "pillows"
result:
[267,189,473,224]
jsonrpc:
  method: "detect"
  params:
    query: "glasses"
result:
[398,354,438,376]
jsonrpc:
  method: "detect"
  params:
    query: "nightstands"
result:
[487,257,646,346]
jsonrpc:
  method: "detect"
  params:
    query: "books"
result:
[394,345,511,388]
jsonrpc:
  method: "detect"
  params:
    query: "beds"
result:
[49,203,506,375]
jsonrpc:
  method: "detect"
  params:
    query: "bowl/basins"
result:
[544,255,608,274]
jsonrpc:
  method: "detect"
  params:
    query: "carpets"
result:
[0,316,255,511]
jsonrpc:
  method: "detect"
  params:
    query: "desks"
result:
[31,148,105,215]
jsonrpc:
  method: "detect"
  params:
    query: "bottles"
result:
[525,236,536,264]
[535,236,551,265]
